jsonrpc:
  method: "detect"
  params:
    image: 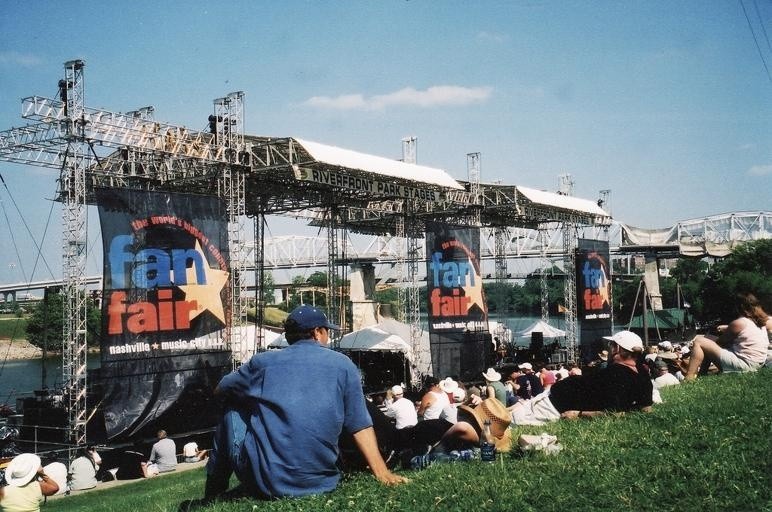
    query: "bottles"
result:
[481,420,497,462]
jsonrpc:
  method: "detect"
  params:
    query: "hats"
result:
[439,376,458,394]
[517,362,533,370]
[676,359,690,374]
[452,387,466,401]
[285,304,340,331]
[601,330,645,353]
[4,452,41,487]
[598,350,609,361]
[391,385,404,397]
[456,397,513,453]
[482,367,502,382]
[658,341,672,349]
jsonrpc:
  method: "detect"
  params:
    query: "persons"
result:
[337,293,771,474]
[177,305,410,511]
[0,429,208,512]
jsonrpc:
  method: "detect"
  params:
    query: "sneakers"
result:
[450,448,475,462]
[409,450,444,473]
